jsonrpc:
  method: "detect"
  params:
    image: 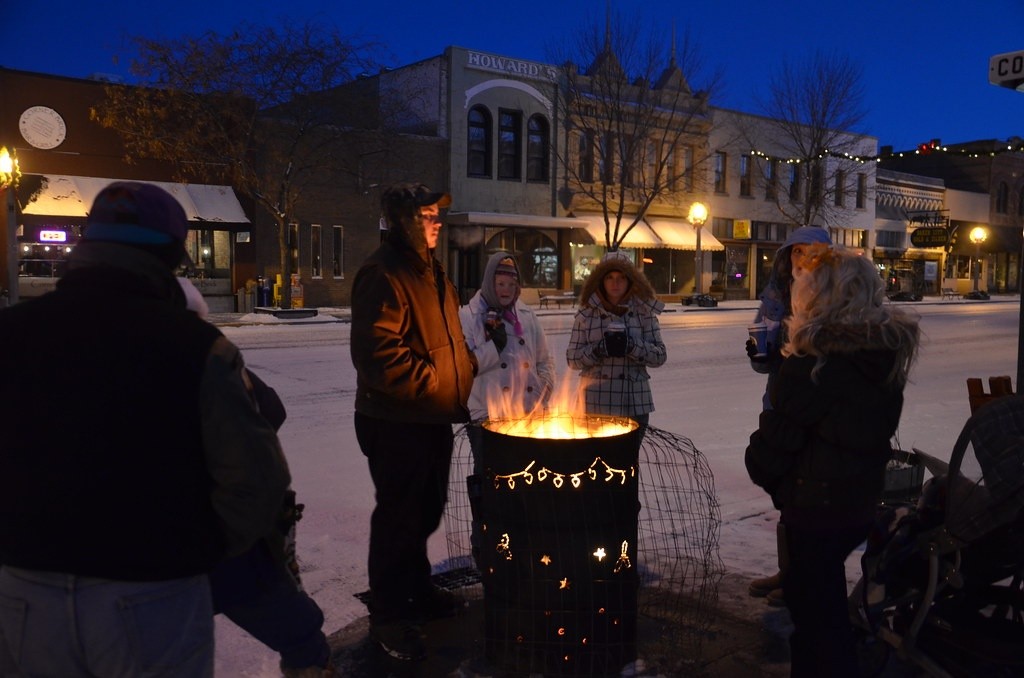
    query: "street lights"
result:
[974,228,984,290]
[692,203,705,294]
[0,155,19,307]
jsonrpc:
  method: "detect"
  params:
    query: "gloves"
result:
[603,331,628,357]
[746,340,771,364]
[482,322,507,353]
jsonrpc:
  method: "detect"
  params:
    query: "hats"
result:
[495,257,518,275]
[87,180,197,272]
[417,185,452,209]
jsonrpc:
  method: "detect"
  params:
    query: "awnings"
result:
[645,216,725,253]
[572,216,664,250]
[16,173,252,223]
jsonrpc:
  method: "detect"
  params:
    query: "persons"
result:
[457,252,555,426]
[749,227,835,599]
[743,247,922,678]
[1,182,296,678]
[349,181,475,660]
[568,257,667,439]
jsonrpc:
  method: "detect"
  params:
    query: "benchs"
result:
[537,288,577,310]
[941,287,961,300]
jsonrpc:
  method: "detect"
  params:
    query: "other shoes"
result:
[369,617,426,659]
[748,575,779,594]
[422,582,471,618]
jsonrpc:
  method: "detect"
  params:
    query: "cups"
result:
[486,308,503,336]
[605,322,627,358]
[748,323,767,356]
